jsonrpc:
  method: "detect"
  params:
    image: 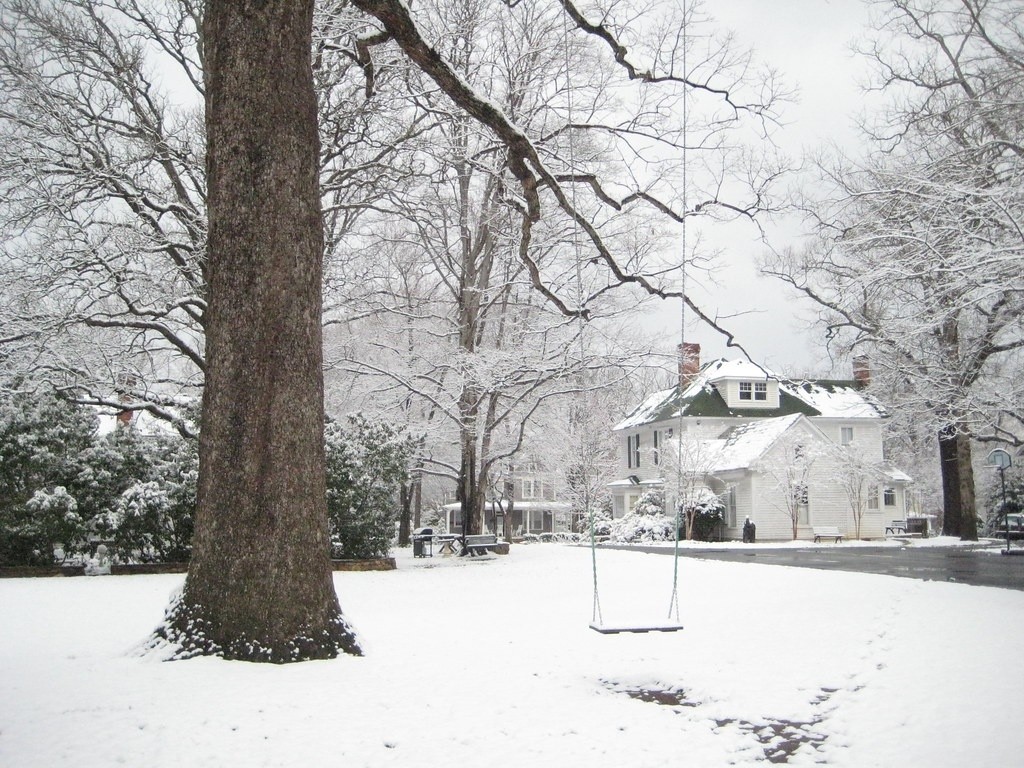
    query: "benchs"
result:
[813,525,844,543]
[465,534,499,556]
[885,522,907,535]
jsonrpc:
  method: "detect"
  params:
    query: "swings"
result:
[559,0,700,635]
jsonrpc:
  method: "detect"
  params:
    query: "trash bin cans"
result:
[412,527,434,557]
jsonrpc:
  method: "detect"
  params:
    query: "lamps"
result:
[628,477,641,485]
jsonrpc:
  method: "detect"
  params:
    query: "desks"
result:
[438,539,455,554]
[437,534,459,554]
